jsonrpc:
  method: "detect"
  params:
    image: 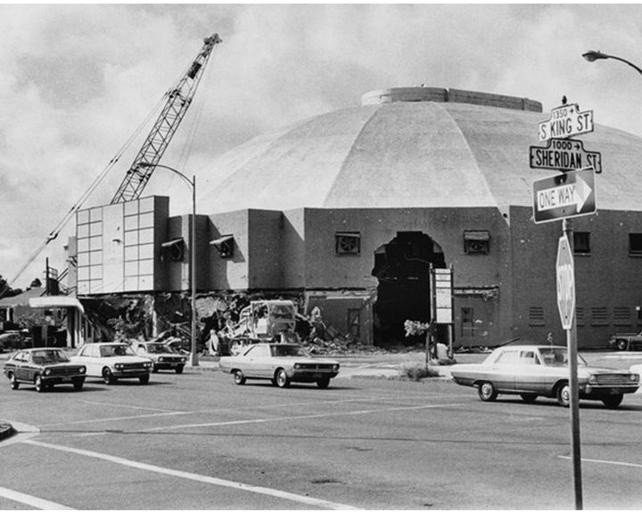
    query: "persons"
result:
[309,302,328,342]
[44,309,59,345]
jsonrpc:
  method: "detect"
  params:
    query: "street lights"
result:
[579,48,642,76]
[135,161,200,368]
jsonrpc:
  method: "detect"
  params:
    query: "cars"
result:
[214,340,342,391]
[606,326,642,351]
[0,327,188,394]
[448,343,642,410]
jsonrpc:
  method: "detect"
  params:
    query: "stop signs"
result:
[552,235,577,332]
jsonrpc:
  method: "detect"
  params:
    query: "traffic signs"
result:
[530,166,597,226]
[527,136,604,174]
[537,103,595,141]
